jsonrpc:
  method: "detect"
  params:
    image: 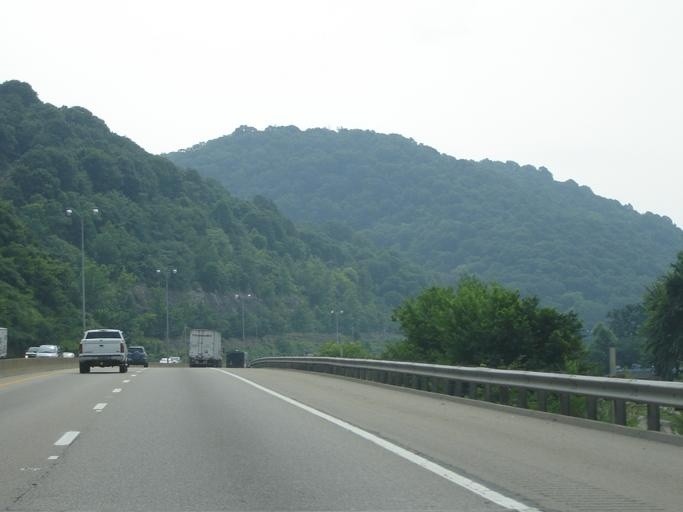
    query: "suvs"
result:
[127,345,148,367]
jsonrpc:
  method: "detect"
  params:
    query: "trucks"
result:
[189,329,223,367]
[226,350,250,368]
[0,328,9,359]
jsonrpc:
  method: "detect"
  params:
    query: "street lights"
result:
[234,293,252,350]
[330,309,343,344]
[65,207,100,337]
[155,268,177,366]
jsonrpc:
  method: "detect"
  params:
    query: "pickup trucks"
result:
[79,328,127,373]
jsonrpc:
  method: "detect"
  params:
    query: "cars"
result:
[159,356,180,363]
[25,345,75,359]
